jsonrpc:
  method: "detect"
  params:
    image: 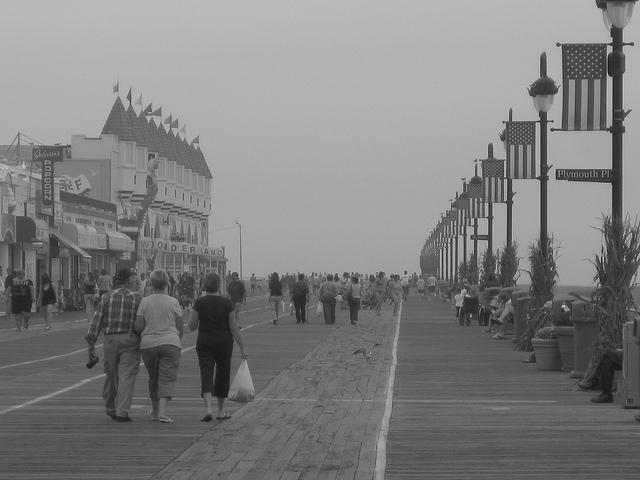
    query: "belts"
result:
[114,332,137,334]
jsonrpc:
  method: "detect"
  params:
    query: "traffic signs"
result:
[555,168,614,182]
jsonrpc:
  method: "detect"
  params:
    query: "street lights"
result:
[525,51,563,247]
[235,220,243,280]
[501,108,515,244]
[419,142,497,276]
[590,0,635,233]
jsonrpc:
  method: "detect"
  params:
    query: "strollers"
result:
[457,287,483,326]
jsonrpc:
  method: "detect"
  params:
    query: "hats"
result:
[113,267,137,282]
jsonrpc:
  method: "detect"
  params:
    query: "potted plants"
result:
[438,219,640,383]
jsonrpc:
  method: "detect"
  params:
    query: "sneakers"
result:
[117,417,132,421]
[577,381,612,403]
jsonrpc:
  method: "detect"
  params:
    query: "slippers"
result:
[147,410,175,423]
[201,410,231,422]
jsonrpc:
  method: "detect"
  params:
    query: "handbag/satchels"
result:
[37,293,44,307]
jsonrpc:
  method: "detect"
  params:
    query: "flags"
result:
[420,183,489,257]
[560,43,608,132]
[480,158,505,204]
[110,78,206,148]
[505,119,536,180]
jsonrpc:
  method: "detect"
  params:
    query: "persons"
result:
[84,266,145,422]
[267,269,438,324]
[127,266,257,329]
[188,272,248,423]
[442,283,514,339]
[133,268,184,423]
[3,268,113,331]
[576,341,624,403]
[521,350,536,363]
